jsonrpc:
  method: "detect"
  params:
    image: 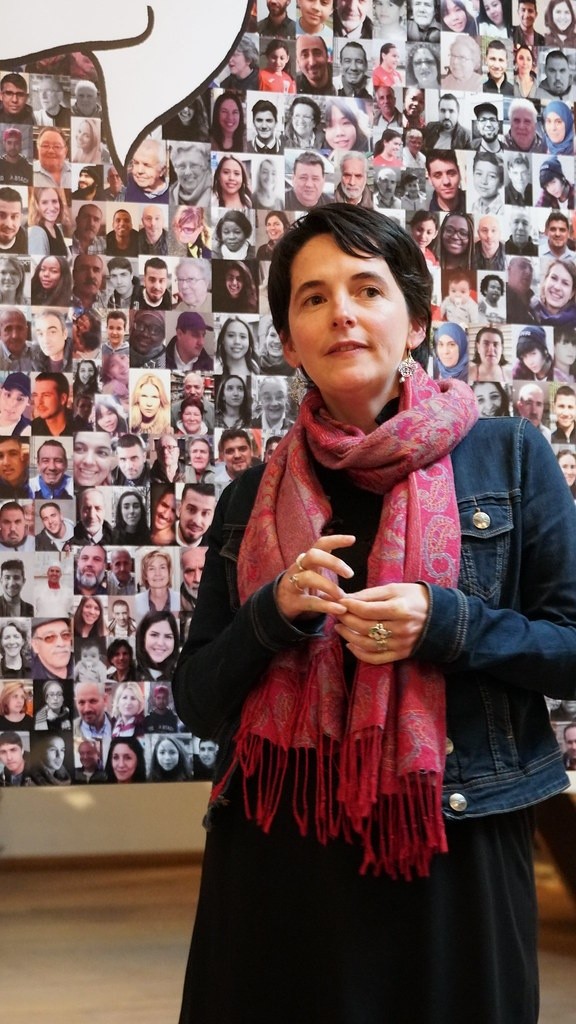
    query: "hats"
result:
[2,372,30,398]
[31,618,71,634]
[175,312,214,332]
[2,127,21,141]
[405,173,419,185]
[153,686,169,698]
[133,310,166,330]
[540,158,562,175]
[520,326,548,347]
[473,101,498,120]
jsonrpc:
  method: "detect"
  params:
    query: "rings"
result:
[368,620,392,640]
[289,573,304,592]
[296,552,306,571]
[376,639,389,652]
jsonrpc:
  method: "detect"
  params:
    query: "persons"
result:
[34,438,72,496]
[1,1,576,787]
[106,739,148,780]
[218,314,256,371]
[169,199,575,1024]
[169,313,217,371]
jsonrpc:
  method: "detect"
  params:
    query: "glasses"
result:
[411,58,437,66]
[46,693,62,698]
[33,633,70,645]
[157,444,179,454]
[2,91,28,97]
[175,276,206,284]
[443,226,469,240]
[132,320,164,337]
[6,693,26,700]
[448,53,475,62]
[174,224,200,234]
